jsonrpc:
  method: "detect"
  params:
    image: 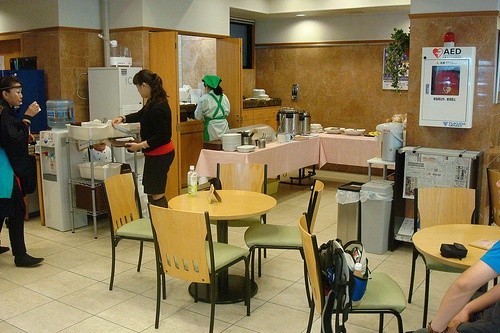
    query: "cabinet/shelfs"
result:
[65,137,139,240]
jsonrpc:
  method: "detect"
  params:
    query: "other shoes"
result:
[0,246,10,254]
[14,253,44,268]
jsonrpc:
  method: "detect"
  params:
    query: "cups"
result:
[258,138,266,147]
[29,101,41,114]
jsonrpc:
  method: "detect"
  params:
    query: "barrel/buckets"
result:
[274,105,311,135]
[380,130,404,161]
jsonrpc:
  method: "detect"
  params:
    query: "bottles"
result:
[35,141,40,154]
[187,166,197,197]
[45,99,67,130]
[67,99,74,125]
[353,263,362,279]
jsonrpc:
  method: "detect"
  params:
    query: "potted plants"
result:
[383,27,411,97]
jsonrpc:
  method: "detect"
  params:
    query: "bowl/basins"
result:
[242,137,252,145]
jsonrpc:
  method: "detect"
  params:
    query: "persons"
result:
[411,241,500,333]
[194,75,230,189]
[112,69,175,208]
[0,76,44,268]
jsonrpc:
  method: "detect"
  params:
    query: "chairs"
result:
[101,162,500,333]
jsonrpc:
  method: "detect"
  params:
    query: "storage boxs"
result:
[75,186,108,211]
[77,162,123,181]
[69,124,130,140]
[263,178,280,194]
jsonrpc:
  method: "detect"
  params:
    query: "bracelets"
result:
[21,115,33,123]
[120,116,124,122]
[427,320,448,333]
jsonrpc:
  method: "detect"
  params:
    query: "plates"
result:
[221,129,309,152]
[309,123,376,138]
[179,82,207,104]
[114,125,130,133]
[247,89,270,100]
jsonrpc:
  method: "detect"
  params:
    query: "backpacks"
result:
[318,239,372,333]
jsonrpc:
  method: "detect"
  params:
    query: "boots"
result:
[153,196,168,209]
[147,195,153,204]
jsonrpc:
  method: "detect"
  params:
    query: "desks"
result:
[193,132,395,194]
[168,189,277,304]
[411,225,500,322]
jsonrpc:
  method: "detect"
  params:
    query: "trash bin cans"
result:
[359,179,395,255]
[337,182,365,245]
